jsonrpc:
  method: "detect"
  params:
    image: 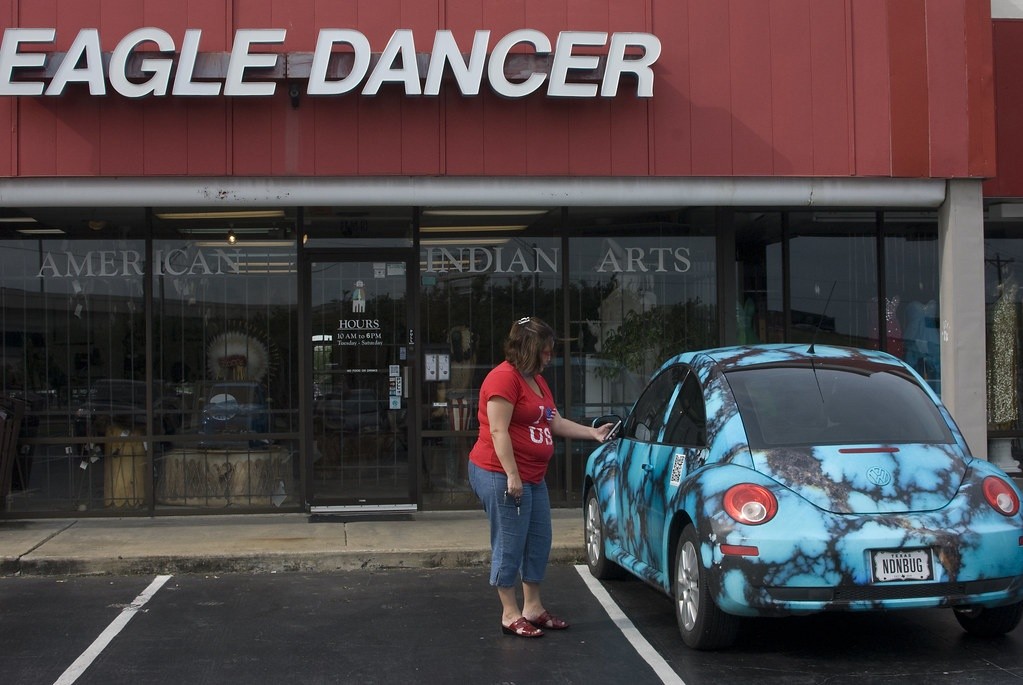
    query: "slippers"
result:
[502,617,543,638]
[527,611,568,629]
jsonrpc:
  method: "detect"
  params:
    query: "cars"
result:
[538,357,648,498]
[197,382,277,448]
[314,368,404,410]
[73,378,180,452]
[420,364,500,433]
[580,343,1023,651]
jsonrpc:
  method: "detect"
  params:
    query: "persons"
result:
[988,264,1019,423]
[904,296,939,367]
[869,286,903,357]
[468,317,616,637]
[736,297,758,345]
[437,331,477,403]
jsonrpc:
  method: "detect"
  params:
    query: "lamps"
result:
[228,230,237,245]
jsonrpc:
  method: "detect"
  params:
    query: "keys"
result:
[503,491,507,504]
[513,498,521,515]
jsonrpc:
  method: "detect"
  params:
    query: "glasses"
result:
[541,349,555,358]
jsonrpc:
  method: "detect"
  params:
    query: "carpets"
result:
[307,514,415,523]
